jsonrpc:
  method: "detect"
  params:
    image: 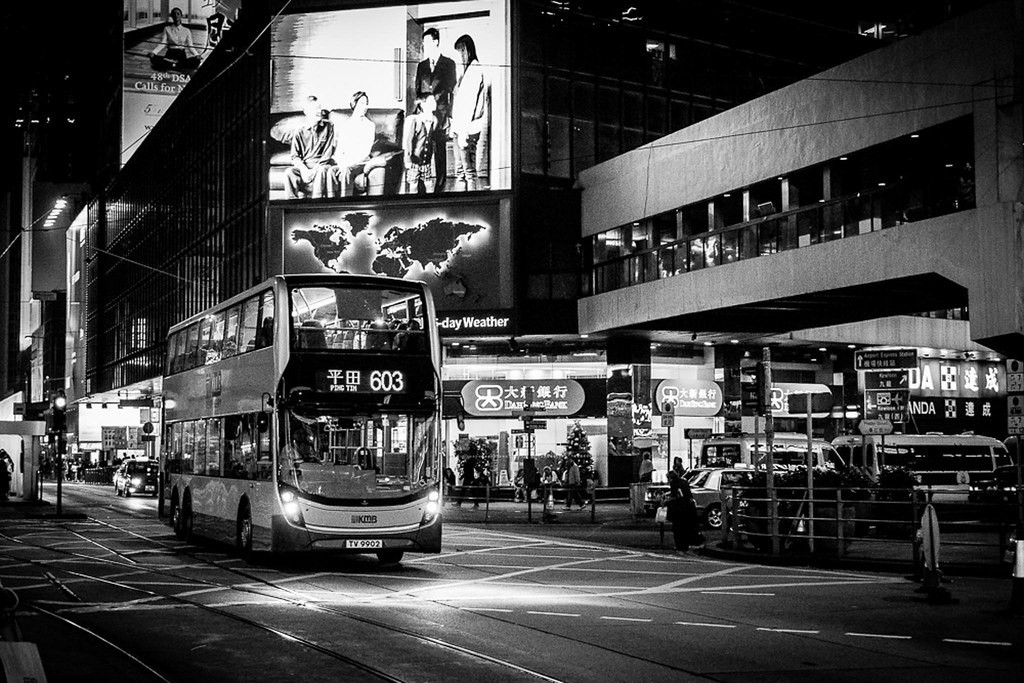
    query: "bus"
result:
[157,273,465,566]
[829,432,1017,506]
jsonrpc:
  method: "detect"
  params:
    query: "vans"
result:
[699,430,851,472]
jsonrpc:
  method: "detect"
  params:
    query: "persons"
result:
[666,457,698,555]
[146,8,203,73]
[261,317,420,350]
[281,428,320,473]
[0,451,160,510]
[512,458,599,513]
[282,27,489,200]
[451,459,480,511]
[640,451,656,483]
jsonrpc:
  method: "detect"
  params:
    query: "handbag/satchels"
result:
[655,503,667,523]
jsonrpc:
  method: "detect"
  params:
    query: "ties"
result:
[430,59,435,74]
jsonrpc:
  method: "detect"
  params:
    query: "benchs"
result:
[444,485,632,514]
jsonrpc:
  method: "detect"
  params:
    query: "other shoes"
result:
[562,506,570,511]
[903,574,921,583]
[578,505,585,510]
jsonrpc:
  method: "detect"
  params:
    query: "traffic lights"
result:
[53,392,67,431]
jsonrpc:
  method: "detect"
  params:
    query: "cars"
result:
[967,464,1024,523]
[113,456,158,498]
[641,463,792,531]
